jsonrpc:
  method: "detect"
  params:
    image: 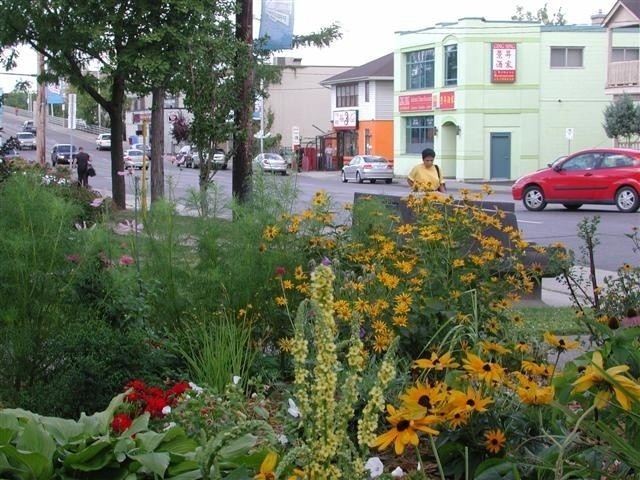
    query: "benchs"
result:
[351,190,572,301]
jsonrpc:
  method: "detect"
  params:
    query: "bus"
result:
[0,87,6,133]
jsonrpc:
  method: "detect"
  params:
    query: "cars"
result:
[340,154,395,184]
[123,142,227,172]
[14,132,36,150]
[50,143,79,166]
[94,132,111,150]
[512,145,640,215]
[251,151,289,176]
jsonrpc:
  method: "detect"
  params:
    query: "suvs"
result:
[22,119,37,136]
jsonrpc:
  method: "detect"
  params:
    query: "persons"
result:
[324,142,334,170]
[406,148,448,198]
[613,158,625,167]
[581,154,596,171]
[72,146,93,189]
[295,144,304,174]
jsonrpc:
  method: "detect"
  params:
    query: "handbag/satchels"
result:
[86,164,96,177]
